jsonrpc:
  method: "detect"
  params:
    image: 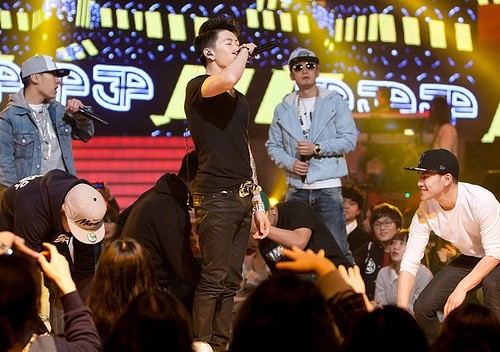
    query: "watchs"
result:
[314,143,323,157]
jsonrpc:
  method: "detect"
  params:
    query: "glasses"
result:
[372,219,395,229]
[292,63,317,72]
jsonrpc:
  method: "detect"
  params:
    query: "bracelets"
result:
[237,46,251,65]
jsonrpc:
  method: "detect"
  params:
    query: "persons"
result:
[83,237,162,352]
[354,202,404,299]
[371,229,434,322]
[337,304,433,352]
[265,45,359,268]
[226,243,373,352]
[354,86,420,195]
[0,168,108,315]
[331,262,380,345]
[0,231,102,352]
[103,285,194,352]
[183,14,272,352]
[0,54,95,192]
[90,182,121,214]
[428,96,459,161]
[334,187,369,265]
[358,203,375,235]
[396,149,500,345]
[112,149,203,314]
[93,202,120,272]
[429,303,488,352]
[249,192,354,280]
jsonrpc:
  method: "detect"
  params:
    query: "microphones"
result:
[77,107,113,128]
[300,154,306,182]
[248,38,282,61]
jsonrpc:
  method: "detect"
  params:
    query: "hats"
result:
[260,191,270,217]
[403,148,459,178]
[20,54,70,79]
[287,47,319,67]
[63,184,107,244]
